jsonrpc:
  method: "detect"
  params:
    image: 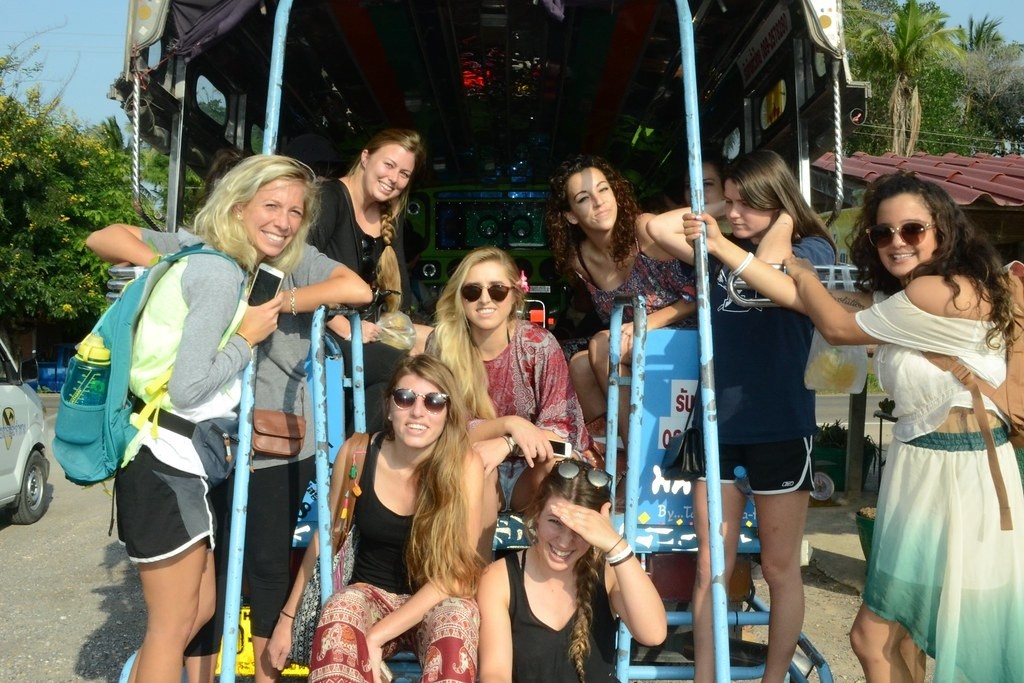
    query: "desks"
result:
[873,410,899,493]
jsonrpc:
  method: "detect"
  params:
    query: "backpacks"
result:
[53,244,247,486]
[926,261,1024,450]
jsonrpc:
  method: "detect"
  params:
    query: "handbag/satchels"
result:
[134,398,238,488]
[571,413,625,491]
[660,427,705,482]
[252,407,307,458]
[289,431,368,665]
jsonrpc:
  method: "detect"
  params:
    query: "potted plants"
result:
[810,419,886,492]
[856,507,876,562]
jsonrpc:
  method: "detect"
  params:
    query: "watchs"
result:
[506,434,518,452]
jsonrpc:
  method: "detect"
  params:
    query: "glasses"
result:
[361,233,376,273]
[557,460,613,493]
[391,387,449,413]
[865,220,936,248]
[461,285,515,302]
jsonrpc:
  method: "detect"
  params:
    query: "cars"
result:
[27,342,78,393]
[1,338,51,526]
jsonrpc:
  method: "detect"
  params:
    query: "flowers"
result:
[515,271,531,296]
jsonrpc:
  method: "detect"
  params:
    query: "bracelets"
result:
[290,286,299,316]
[501,435,512,452]
[605,544,632,563]
[610,551,633,567]
[731,251,754,276]
[345,334,351,341]
[607,537,623,554]
[280,609,295,620]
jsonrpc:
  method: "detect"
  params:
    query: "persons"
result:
[80,124,839,683]
[683,170,1023,683]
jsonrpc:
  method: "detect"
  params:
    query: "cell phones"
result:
[247,263,285,307]
[548,440,572,457]
